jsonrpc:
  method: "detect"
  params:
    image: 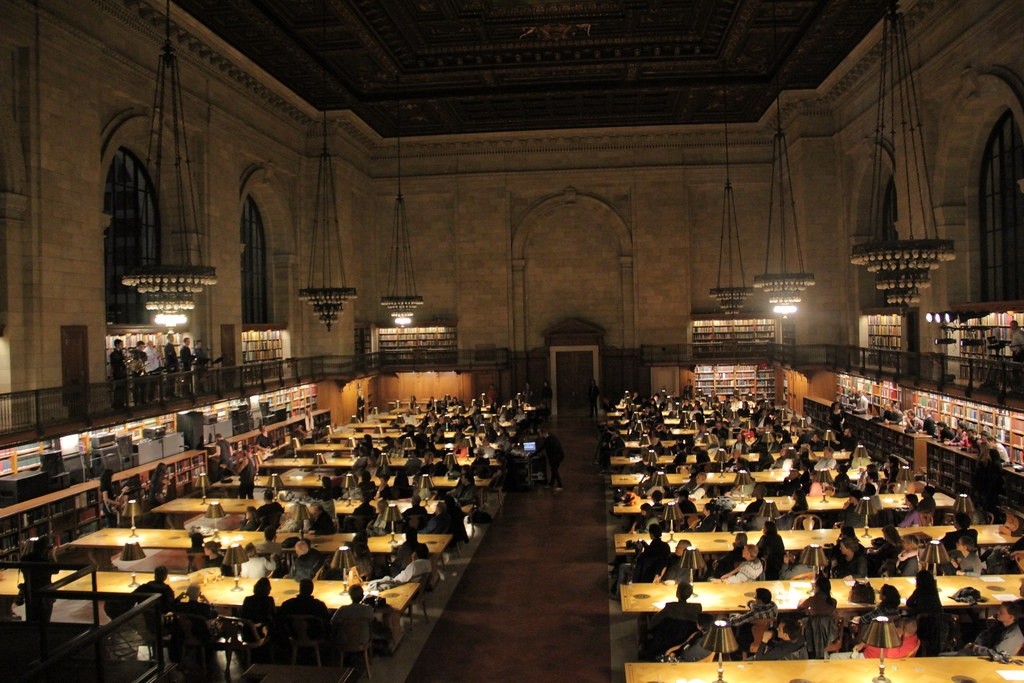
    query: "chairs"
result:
[153,612,378,678]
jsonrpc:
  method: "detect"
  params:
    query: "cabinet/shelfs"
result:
[943,307,1024,386]
[353,320,458,368]
[691,362,1023,523]
[0,329,317,565]
[691,315,916,370]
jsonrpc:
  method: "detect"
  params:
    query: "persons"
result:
[976,431,1004,518]
[610,471,1024,663]
[131,471,479,664]
[1010,320,1024,390]
[110,334,210,411]
[213,423,273,501]
[20,536,60,622]
[100,463,176,531]
[590,378,901,481]
[877,402,977,453]
[355,380,566,493]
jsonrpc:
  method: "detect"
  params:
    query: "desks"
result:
[604,402,1024,682]
[0,398,539,635]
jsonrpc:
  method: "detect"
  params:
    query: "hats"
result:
[915,569,936,589]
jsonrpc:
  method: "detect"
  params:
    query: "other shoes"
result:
[553,487,564,491]
[544,485,553,489]
[600,469,607,474]
[592,462,600,466]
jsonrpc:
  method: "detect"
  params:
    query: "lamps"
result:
[680,545,706,597]
[920,539,950,591]
[119,541,147,587]
[122,500,142,538]
[330,545,356,595]
[383,504,403,544]
[700,620,740,682]
[862,616,902,683]
[801,543,829,594]
[205,502,225,539]
[222,543,249,593]
[120,0,955,327]
[926,311,988,346]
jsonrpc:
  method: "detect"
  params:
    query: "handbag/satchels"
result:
[848,579,875,604]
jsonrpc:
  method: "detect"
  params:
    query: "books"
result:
[868,315,901,366]
[783,319,795,360]
[242,333,283,383]
[177,455,206,498]
[355,329,458,364]
[837,376,901,416]
[927,445,972,500]
[906,389,1024,464]
[960,312,1023,381]
[804,400,914,463]
[693,320,775,357]
[261,384,319,420]
[0,490,99,565]
[695,364,775,405]
